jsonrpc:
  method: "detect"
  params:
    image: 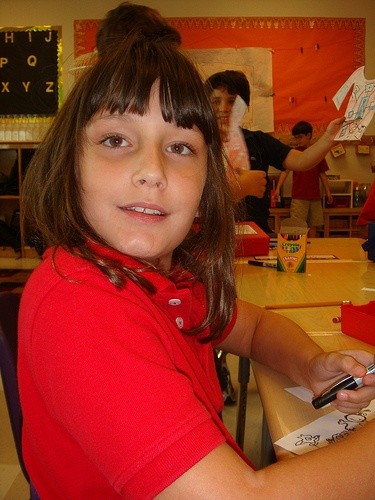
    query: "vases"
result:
[354,190,367,207]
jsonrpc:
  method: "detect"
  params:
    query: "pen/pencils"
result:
[259,300,351,308]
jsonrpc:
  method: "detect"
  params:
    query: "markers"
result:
[248,261,277,268]
[312,364,375,409]
[333,316,341,323]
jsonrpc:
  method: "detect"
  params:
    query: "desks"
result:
[248,305,375,458]
[230,237,375,453]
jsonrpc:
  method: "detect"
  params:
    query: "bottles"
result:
[0,114,55,140]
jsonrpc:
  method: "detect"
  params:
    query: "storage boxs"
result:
[340,299,375,346]
[277,218,310,273]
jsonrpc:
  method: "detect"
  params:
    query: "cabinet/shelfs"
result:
[325,180,354,208]
[323,207,369,238]
[0,143,62,257]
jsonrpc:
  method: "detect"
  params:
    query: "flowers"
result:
[354,181,368,191]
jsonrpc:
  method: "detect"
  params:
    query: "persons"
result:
[272,121,332,238]
[205,71,349,235]
[17,5,375,499]
